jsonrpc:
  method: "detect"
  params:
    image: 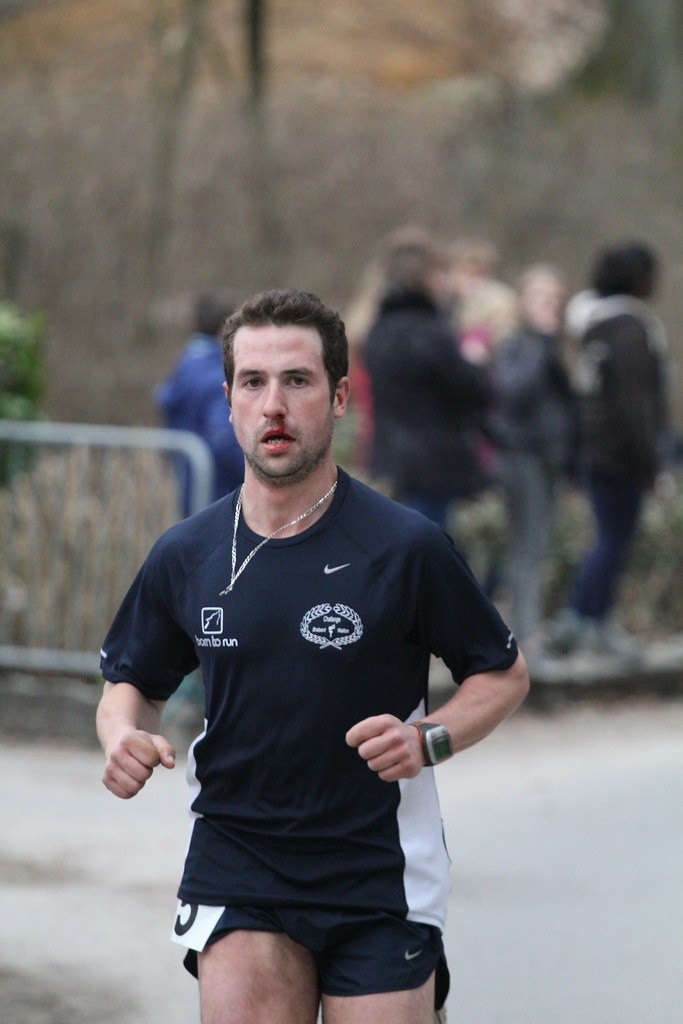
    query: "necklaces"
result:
[218,481,339,597]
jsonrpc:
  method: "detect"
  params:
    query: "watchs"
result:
[410,719,454,766]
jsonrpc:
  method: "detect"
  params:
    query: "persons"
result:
[345,225,682,670]
[152,296,245,516]
[93,290,531,1024]
